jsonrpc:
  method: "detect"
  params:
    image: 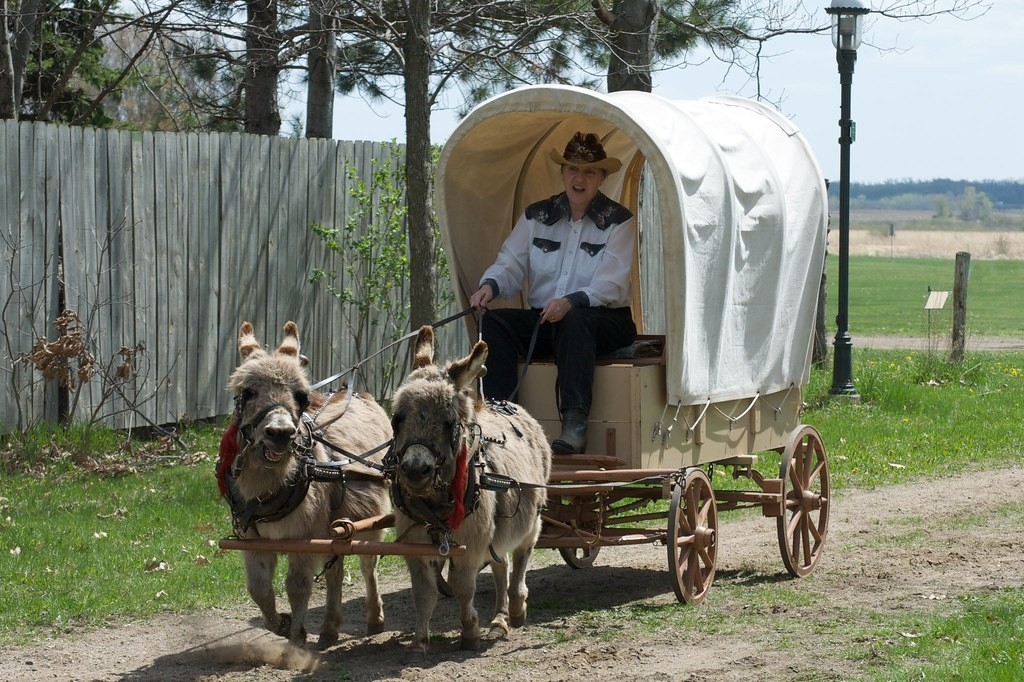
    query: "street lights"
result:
[824,0,870,396]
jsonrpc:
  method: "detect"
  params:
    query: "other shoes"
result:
[551,408,588,454]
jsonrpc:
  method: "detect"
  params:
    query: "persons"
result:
[470,131,637,456]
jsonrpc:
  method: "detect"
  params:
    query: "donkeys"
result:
[217,322,553,666]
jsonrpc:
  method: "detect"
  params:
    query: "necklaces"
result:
[571,214,581,221]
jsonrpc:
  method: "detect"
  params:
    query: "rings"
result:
[551,317,555,320]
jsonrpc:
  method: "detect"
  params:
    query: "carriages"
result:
[211,83,833,668]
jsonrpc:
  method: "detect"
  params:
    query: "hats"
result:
[550,131,622,176]
